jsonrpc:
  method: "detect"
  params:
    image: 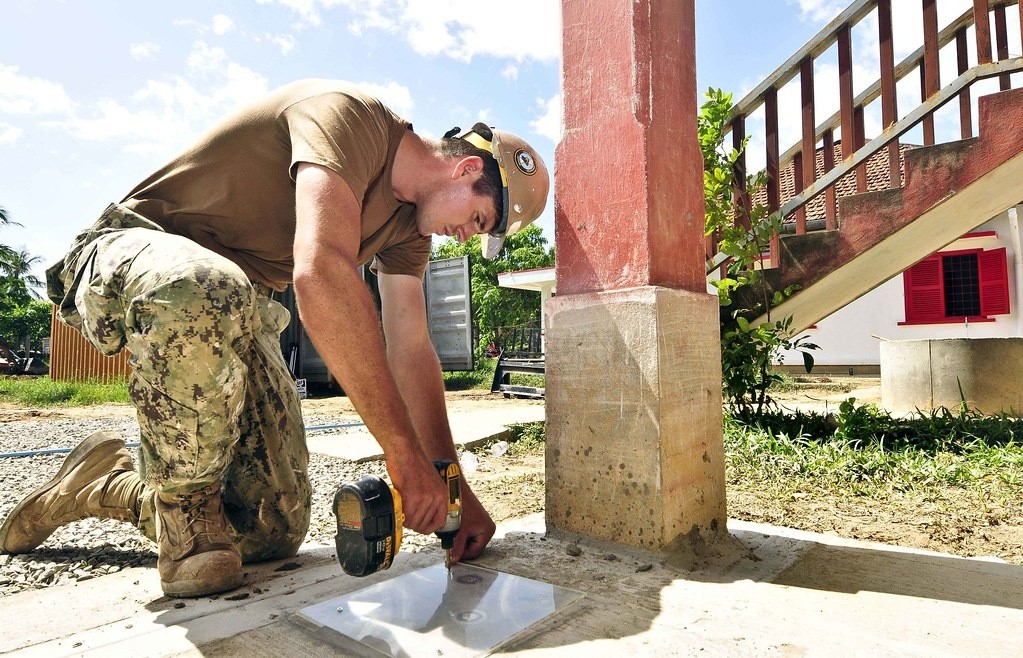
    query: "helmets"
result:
[472,122,550,259]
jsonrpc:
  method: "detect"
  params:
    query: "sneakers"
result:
[0,430,144,555]
[154,491,243,598]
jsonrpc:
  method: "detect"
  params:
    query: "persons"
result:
[0,78,551,596]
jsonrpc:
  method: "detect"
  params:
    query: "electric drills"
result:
[333,460,463,577]
